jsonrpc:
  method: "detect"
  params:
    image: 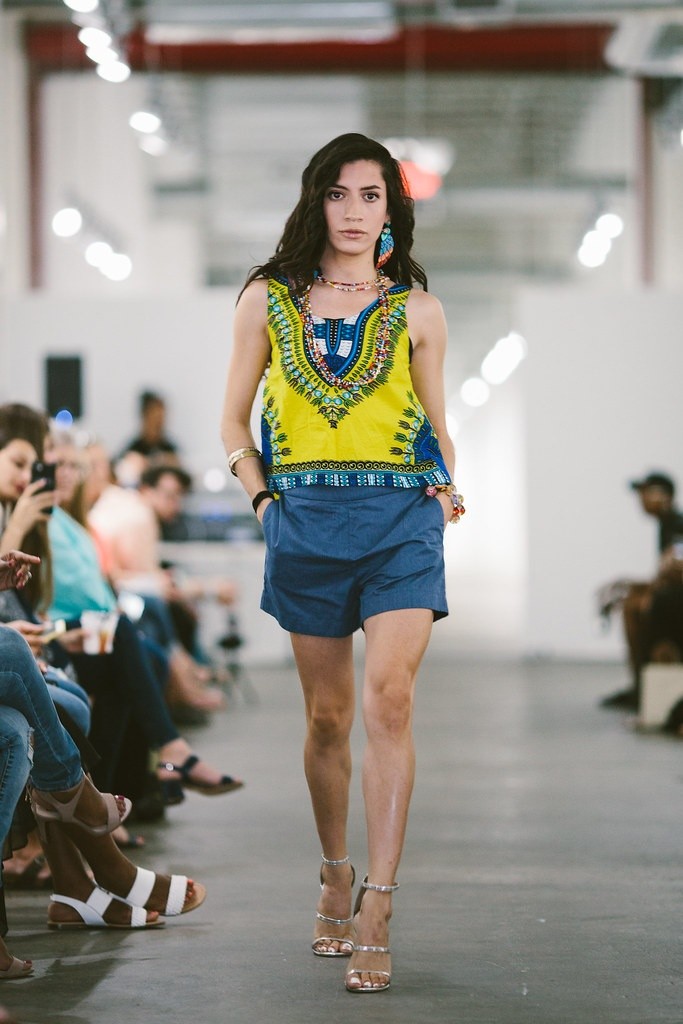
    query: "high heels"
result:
[154,754,242,804]
[30,772,132,844]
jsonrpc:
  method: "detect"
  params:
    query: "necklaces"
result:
[294,268,390,386]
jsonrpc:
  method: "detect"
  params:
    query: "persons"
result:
[602,473,683,733]
[219,133,466,992]
[0,392,242,977]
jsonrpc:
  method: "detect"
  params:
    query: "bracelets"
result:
[426,484,466,523]
[229,447,262,477]
[252,491,275,514]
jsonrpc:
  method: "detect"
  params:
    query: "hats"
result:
[632,472,674,497]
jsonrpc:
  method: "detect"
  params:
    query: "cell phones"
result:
[31,461,56,514]
[40,620,66,643]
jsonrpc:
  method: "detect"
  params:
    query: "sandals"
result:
[45,886,166,928]
[112,824,146,849]
[311,853,356,957]
[0,954,35,978]
[343,874,399,993]
[95,865,207,915]
